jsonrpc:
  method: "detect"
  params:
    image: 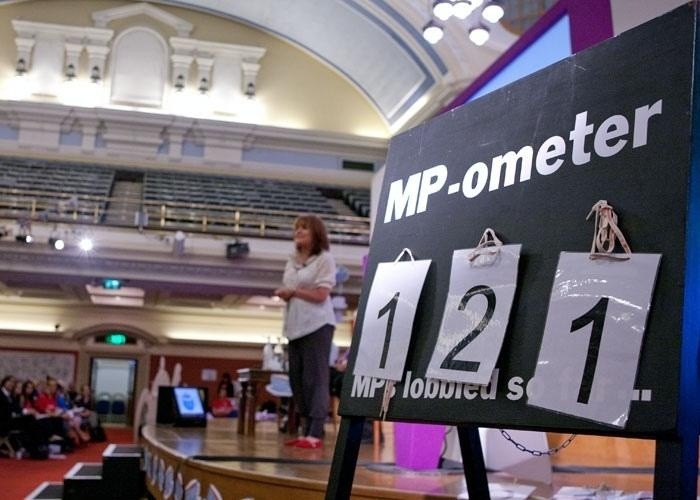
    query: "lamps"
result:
[468,23,489,46]
[48,238,63,249]
[64,64,75,81]
[90,64,100,82]
[15,59,26,76]
[480,4,503,22]
[175,74,185,90]
[452,0,472,19]
[245,83,255,98]
[423,19,444,43]
[16,235,34,244]
[197,77,208,93]
[432,1,453,20]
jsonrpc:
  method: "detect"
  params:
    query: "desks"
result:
[235,369,299,435]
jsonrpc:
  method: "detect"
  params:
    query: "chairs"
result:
[1,155,116,223]
[142,168,343,232]
[341,186,370,216]
[0,430,20,457]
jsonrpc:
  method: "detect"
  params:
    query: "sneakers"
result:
[283,437,305,448]
[292,439,324,451]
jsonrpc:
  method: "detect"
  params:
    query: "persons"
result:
[0,373,103,459]
[273,214,337,447]
[216,372,234,397]
[328,341,342,430]
